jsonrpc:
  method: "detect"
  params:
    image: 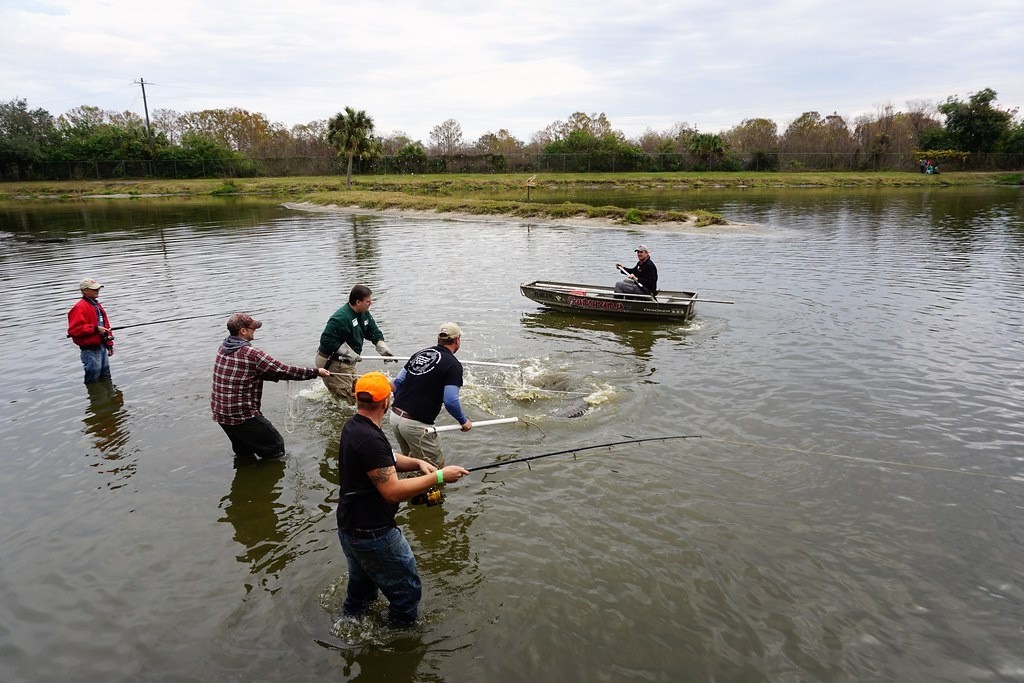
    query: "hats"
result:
[227,313,262,329]
[79,278,104,290]
[437,322,460,340]
[634,244,649,251]
[355,371,391,402]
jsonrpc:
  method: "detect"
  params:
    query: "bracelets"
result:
[436,469,443,483]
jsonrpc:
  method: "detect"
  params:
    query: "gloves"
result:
[375,340,398,364]
[336,341,363,364]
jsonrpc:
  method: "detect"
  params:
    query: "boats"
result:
[521,280,699,324]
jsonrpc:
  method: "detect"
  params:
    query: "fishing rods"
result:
[67,308,231,341]
[410,433,706,509]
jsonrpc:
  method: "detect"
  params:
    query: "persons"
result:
[67,278,114,384]
[336,372,470,622]
[211,314,331,457]
[316,285,396,399]
[389,322,472,469]
[614,245,658,300]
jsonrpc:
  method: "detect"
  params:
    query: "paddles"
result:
[617,265,658,303]
